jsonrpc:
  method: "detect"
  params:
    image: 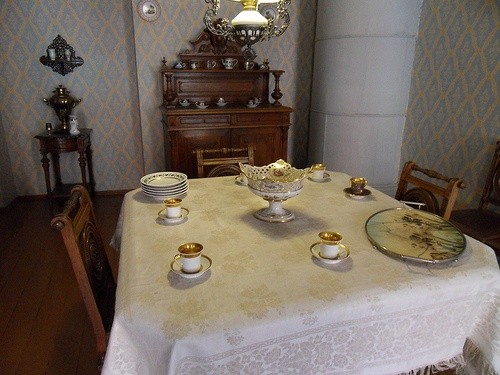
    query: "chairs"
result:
[393,161,467,223]
[50,183,115,366]
[192,146,256,179]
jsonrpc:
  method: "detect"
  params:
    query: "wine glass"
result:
[46,123,52,136]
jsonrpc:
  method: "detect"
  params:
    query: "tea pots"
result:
[175,57,254,70]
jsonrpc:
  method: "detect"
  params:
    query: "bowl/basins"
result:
[196,105,208,109]
[181,103,190,106]
[247,104,257,108]
[216,103,227,106]
[254,102,262,104]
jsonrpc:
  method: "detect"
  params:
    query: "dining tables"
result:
[102,170,500,375]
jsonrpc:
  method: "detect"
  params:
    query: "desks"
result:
[34,128,96,207]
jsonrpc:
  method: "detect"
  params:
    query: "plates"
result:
[170,255,212,278]
[308,172,331,182]
[235,175,248,186]
[157,206,190,223]
[140,172,189,201]
[344,188,371,199]
[308,241,350,266]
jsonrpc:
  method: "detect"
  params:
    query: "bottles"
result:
[69,115,80,135]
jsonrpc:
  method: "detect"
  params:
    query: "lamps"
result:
[202,0,291,61]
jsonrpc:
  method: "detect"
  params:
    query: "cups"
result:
[174,242,203,273]
[350,177,367,194]
[311,164,325,179]
[318,230,343,259]
[240,172,248,182]
[163,198,182,218]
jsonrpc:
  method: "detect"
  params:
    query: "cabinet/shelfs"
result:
[158,18,293,177]
